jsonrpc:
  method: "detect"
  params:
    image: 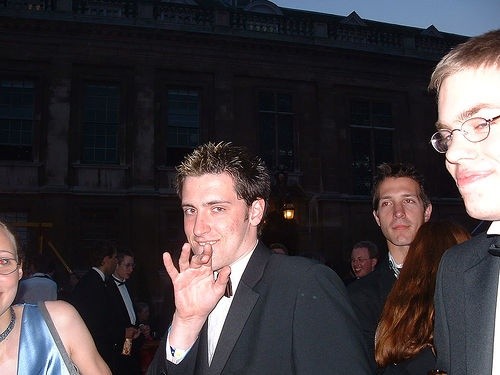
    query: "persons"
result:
[374,217,473,375]
[104,249,151,375]
[10,256,57,307]
[269,244,290,256]
[73,240,121,375]
[0,221,112,375]
[146,142,381,375]
[430,27,499,375]
[342,242,380,289]
[345,163,433,374]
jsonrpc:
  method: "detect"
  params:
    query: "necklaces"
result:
[0,306,16,342]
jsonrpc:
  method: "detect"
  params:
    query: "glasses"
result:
[431,115,500,154]
[0,258,21,275]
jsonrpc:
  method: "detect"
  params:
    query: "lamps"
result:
[279,196,297,225]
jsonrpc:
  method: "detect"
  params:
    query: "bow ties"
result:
[112,277,126,286]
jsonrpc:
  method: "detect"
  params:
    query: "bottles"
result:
[121,326,133,356]
